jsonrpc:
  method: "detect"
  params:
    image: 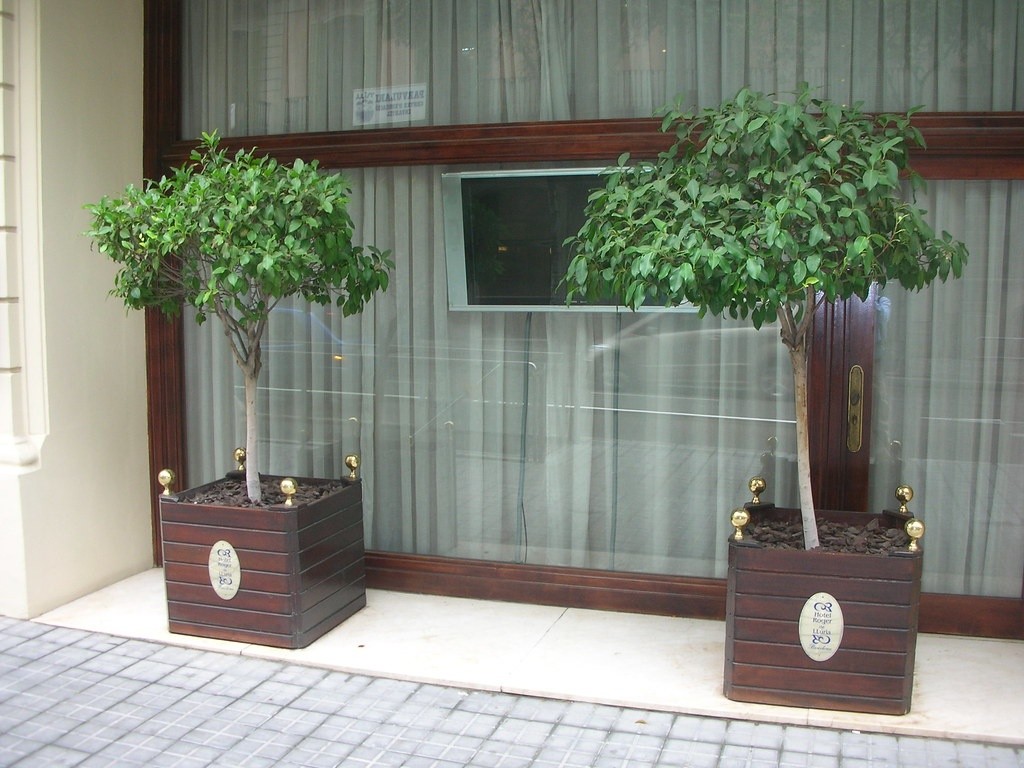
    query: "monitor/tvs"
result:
[439,166,702,312]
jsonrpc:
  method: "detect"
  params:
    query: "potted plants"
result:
[552,80,969,715]
[80,126,396,650]
